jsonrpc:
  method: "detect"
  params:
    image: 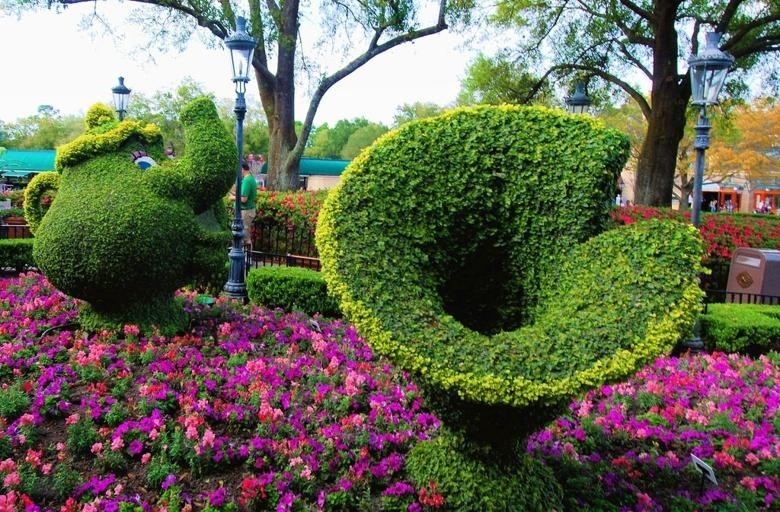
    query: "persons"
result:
[228,162,257,266]
[616,186,775,217]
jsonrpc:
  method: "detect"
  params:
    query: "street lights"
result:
[111,73,132,122]
[683,31,734,356]
[565,79,592,118]
[214,14,262,302]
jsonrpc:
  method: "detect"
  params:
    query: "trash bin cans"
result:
[725,248,780,305]
